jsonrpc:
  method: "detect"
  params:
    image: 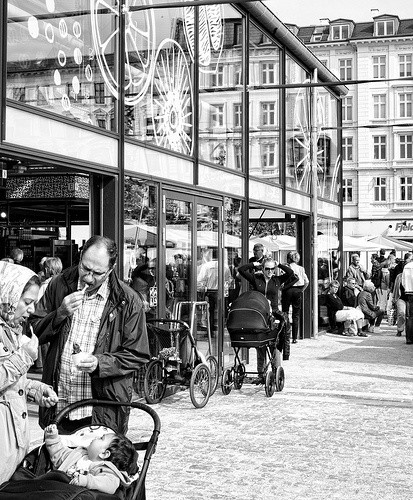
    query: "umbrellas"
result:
[124,224,413,279]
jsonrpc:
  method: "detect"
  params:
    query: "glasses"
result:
[80,250,110,280]
[265,267,275,271]
[352,283,356,285]
[254,249,260,252]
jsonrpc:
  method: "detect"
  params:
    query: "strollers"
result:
[0,397,162,500]
[219,290,290,397]
[142,316,220,409]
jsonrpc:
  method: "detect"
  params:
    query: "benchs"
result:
[344,319,369,336]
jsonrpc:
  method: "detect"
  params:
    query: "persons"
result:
[0,244,413,344]
[239,258,299,384]
[0,262,58,483]
[26,236,151,436]
[44,424,136,495]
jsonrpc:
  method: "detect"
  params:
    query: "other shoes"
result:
[397,332,401,336]
[292,340,297,343]
[358,330,369,337]
[369,327,383,334]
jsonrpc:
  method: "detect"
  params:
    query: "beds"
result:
[226,328,278,347]
